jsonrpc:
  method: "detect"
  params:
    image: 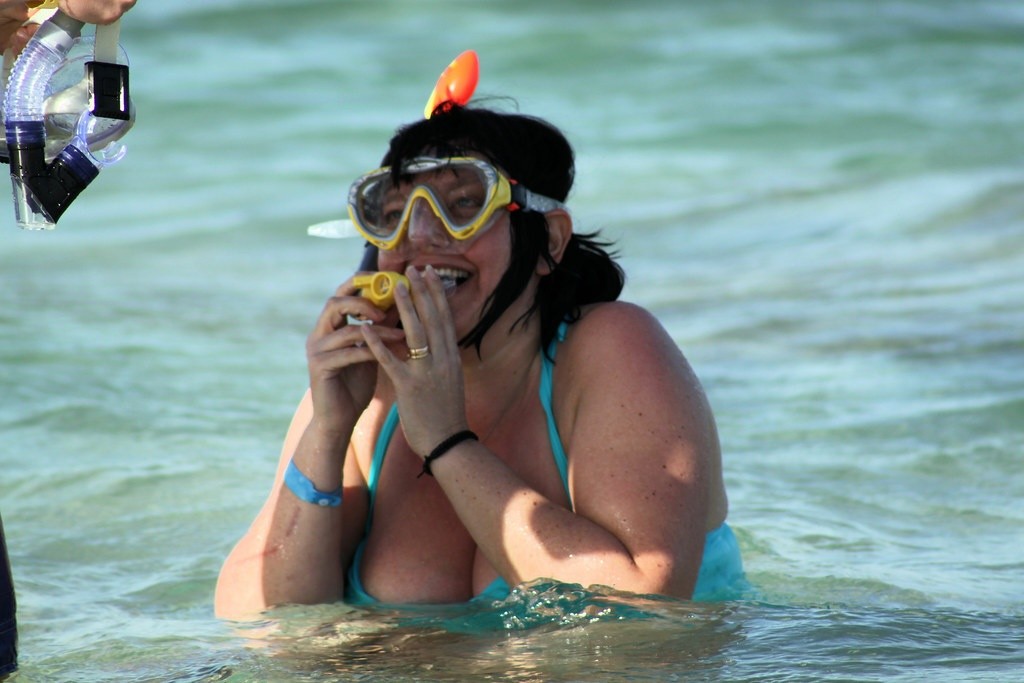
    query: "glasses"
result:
[348,156,566,251]
[0,36,135,164]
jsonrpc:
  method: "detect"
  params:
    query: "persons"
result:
[213,89,752,626]
[0,0,138,682]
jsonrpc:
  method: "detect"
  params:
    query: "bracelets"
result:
[282,460,345,507]
[415,428,479,479]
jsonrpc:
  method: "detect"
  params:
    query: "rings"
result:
[406,344,429,359]
